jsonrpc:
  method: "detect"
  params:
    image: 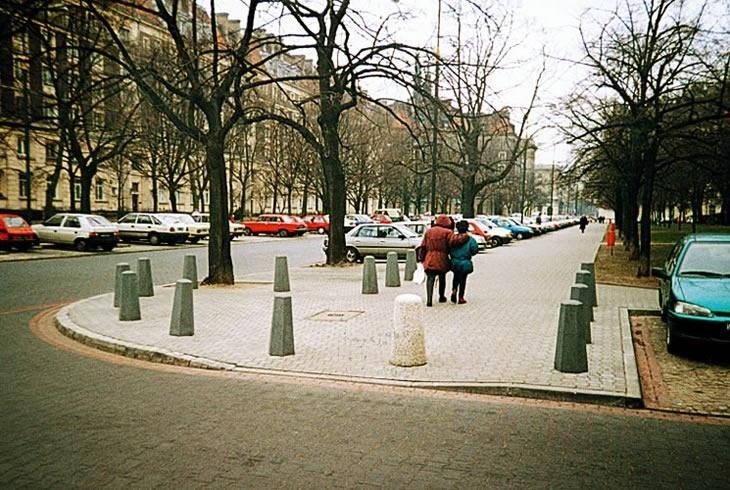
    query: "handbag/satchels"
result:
[415,246,425,262]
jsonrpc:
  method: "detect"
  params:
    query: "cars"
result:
[191,209,246,243]
[0,212,36,255]
[648,230,730,354]
[175,212,210,246]
[107,211,188,247]
[28,212,121,253]
[320,221,425,264]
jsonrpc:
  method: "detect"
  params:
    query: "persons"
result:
[450,220,478,303]
[579,214,588,233]
[536,213,541,225]
[419,215,472,307]
[598,216,605,224]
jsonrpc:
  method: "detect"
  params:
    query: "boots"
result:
[427,296,432,306]
[439,293,446,302]
[459,296,466,303]
[451,288,457,303]
